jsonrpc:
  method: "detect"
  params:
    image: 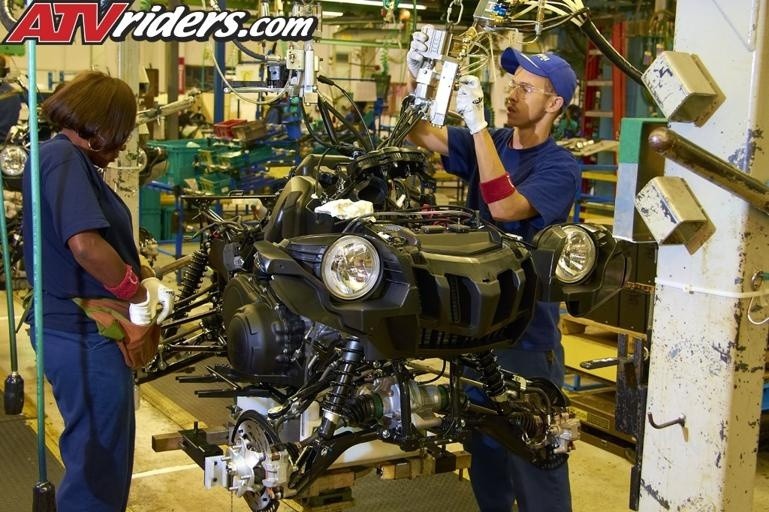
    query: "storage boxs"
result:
[139,119,272,241]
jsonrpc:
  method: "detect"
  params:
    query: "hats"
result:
[501,46,578,105]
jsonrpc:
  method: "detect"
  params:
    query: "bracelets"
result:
[477,170,515,204]
[102,264,140,302]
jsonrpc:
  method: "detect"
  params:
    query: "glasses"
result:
[507,78,554,100]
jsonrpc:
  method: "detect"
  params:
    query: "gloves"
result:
[456,75,489,135]
[140,277,175,325]
[128,288,151,326]
[406,31,429,79]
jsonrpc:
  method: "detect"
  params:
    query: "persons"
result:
[21,70,174,511]
[405,23,582,512]
[0,55,22,143]
